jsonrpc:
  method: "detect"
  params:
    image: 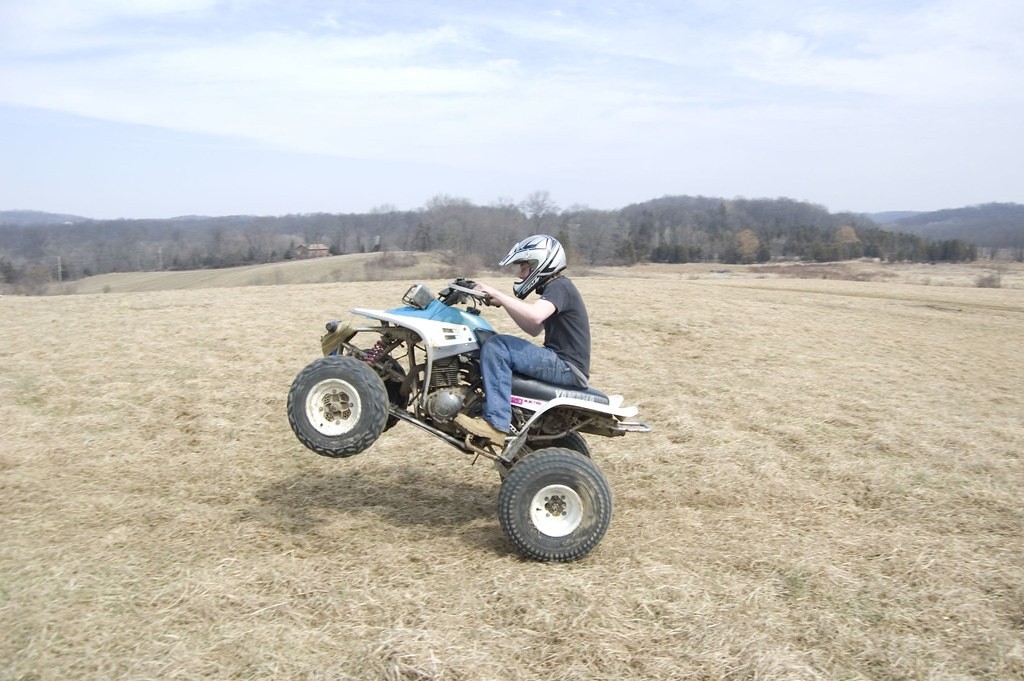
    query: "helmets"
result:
[498,234,568,300]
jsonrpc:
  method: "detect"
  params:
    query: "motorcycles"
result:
[285,280,650,562]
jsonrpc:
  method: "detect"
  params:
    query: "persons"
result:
[452,233,591,448]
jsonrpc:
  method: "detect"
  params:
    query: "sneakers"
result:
[453,412,507,447]
